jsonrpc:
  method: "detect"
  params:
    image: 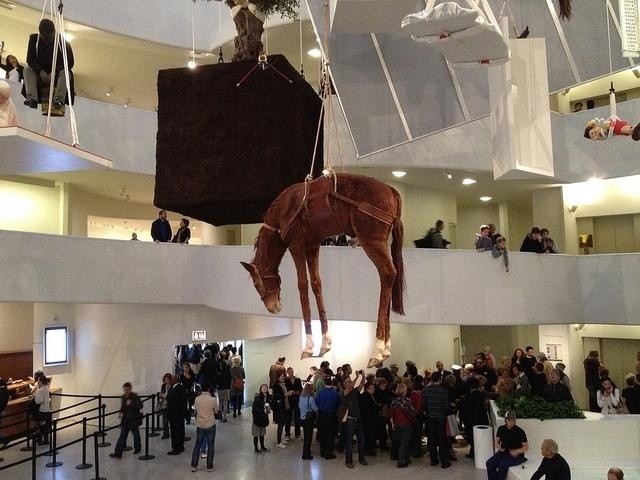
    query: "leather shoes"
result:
[24,100,37,108]
[52,100,62,109]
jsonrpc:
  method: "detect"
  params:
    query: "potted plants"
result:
[154,0,324,226]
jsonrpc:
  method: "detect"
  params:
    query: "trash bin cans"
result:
[473,424,494,470]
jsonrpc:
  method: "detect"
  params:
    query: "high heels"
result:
[304,410,316,426]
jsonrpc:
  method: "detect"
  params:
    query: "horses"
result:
[239,173,406,368]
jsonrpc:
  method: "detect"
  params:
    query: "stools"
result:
[40,82,65,116]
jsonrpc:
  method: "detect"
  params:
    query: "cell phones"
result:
[358,371,362,374]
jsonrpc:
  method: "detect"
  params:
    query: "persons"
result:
[474,224,558,272]
[321,234,363,247]
[586,100,595,109]
[21,19,74,108]
[428,220,446,248]
[108,382,144,459]
[0,377,9,445]
[151,210,172,243]
[28,371,44,393]
[573,102,583,112]
[252,343,585,468]
[485,411,529,480]
[0,41,24,83]
[582,350,639,414]
[607,466,624,479]
[531,439,571,479]
[32,375,51,445]
[131,233,138,240]
[157,343,246,473]
[171,218,190,244]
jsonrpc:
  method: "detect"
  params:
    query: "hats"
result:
[555,363,565,372]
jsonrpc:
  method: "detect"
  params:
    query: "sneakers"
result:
[110,453,122,457]
[192,466,197,472]
[347,463,353,468]
[208,465,216,471]
[253,434,303,454]
[391,455,457,468]
[303,453,337,460]
[201,452,208,458]
[465,453,474,459]
[161,434,169,439]
[133,447,141,454]
[360,460,367,465]
[168,446,184,455]
[232,412,241,417]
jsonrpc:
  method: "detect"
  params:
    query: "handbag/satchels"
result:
[446,414,460,438]
[232,375,244,391]
[28,397,40,414]
[215,410,222,419]
[616,404,630,413]
